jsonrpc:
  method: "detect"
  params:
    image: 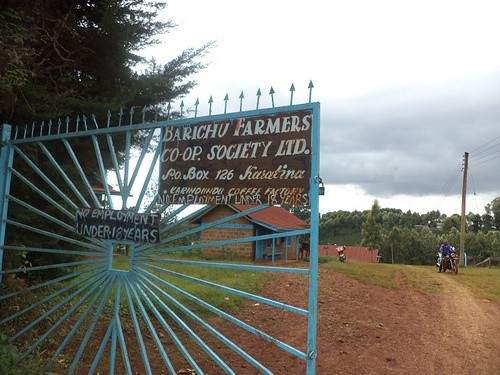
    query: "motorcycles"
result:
[437,251,458,275]
[334,244,345,263]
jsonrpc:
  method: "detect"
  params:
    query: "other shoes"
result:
[438,270,441,273]
[443,269,446,272]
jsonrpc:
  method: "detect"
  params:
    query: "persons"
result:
[437,240,452,273]
